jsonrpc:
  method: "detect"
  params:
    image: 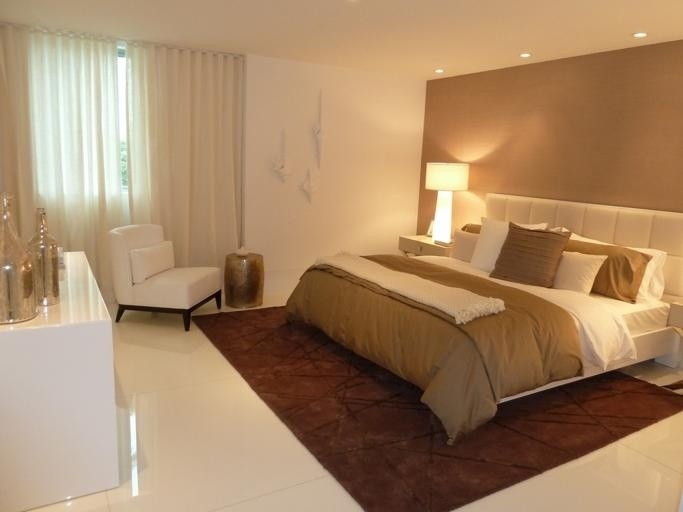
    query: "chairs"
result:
[109,223,225,332]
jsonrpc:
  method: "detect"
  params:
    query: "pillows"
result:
[468,213,549,282]
[551,247,609,296]
[552,226,574,239]
[490,221,573,291]
[129,239,176,284]
[565,237,670,304]
[450,227,480,262]
[461,223,480,233]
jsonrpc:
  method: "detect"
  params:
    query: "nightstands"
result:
[399,229,454,258]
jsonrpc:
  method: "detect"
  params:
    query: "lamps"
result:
[425,163,470,245]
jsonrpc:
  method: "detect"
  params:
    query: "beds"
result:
[284,193,682,448]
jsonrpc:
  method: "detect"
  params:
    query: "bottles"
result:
[28,208,60,306]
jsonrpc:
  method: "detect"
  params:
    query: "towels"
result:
[309,247,508,325]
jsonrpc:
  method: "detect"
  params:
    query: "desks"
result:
[0,250,121,512]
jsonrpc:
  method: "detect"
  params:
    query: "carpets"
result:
[192,305,682,512]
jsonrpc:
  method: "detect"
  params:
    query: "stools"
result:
[226,252,265,309]
